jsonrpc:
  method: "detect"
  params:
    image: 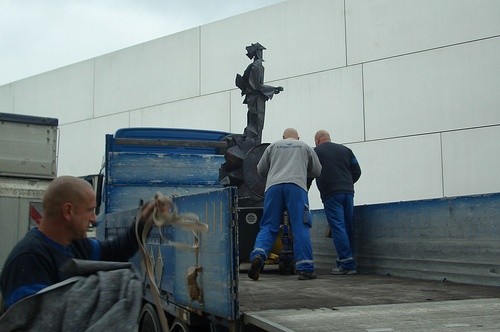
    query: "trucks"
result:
[80,127,500,331]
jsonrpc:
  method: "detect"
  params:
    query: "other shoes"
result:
[248,258,264,281]
[298,271,317,280]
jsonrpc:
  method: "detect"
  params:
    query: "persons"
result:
[0,173,166,332]
[247,129,322,280]
[307,130,362,273]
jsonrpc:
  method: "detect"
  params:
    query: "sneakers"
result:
[330,265,357,274]
